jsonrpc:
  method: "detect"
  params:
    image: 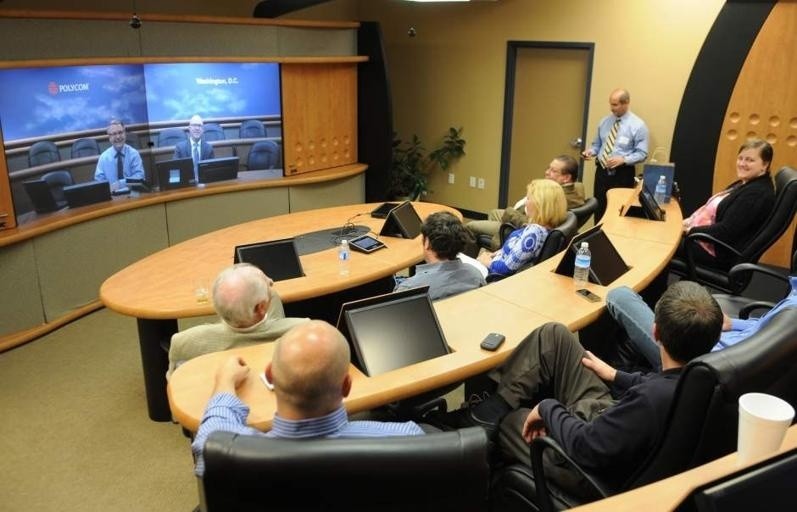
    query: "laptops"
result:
[21,180,68,215]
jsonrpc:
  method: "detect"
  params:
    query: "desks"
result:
[99,201,462,421]
[166,188,683,433]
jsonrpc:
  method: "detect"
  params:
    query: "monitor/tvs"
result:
[235,240,305,282]
[638,186,662,221]
[391,202,423,239]
[570,229,629,287]
[0,63,153,227]
[142,55,285,192]
[62,181,112,209]
[154,158,195,191]
[197,157,239,183]
[343,292,450,378]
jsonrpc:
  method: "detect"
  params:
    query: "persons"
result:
[94,120,146,193]
[478,179,567,275]
[605,274,797,371]
[173,116,215,181]
[584,90,649,227]
[642,142,775,299]
[466,156,584,237]
[169,266,310,365]
[190,320,423,476]
[427,281,722,491]
[392,213,487,301]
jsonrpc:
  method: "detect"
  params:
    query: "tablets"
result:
[347,234,384,254]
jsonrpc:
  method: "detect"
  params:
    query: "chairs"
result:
[124,133,141,151]
[71,139,98,158]
[202,122,225,141]
[247,142,281,170]
[494,305,796,512]
[711,224,796,320]
[239,120,265,139]
[476,196,598,247]
[475,211,576,283]
[158,128,187,146]
[668,166,796,296]
[29,141,60,167]
[190,425,495,511]
[41,170,72,201]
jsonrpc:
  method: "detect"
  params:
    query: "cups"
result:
[738,392,795,468]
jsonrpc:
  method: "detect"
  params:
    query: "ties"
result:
[601,120,620,170]
[115,151,124,181]
[193,143,200,179]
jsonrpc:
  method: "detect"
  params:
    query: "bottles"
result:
[654,174,667,207]
[572,241,591,290]
[338,240,350,277]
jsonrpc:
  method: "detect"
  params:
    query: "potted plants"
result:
[383,126,466,200]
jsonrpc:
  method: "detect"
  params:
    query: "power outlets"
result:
[448,173,454,183]
[470,177,476,187]
[478,178,485,188]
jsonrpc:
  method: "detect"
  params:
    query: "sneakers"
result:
[425,405,500,439]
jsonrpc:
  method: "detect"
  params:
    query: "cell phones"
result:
[574,289,601,303]
[479,333,505,352]
